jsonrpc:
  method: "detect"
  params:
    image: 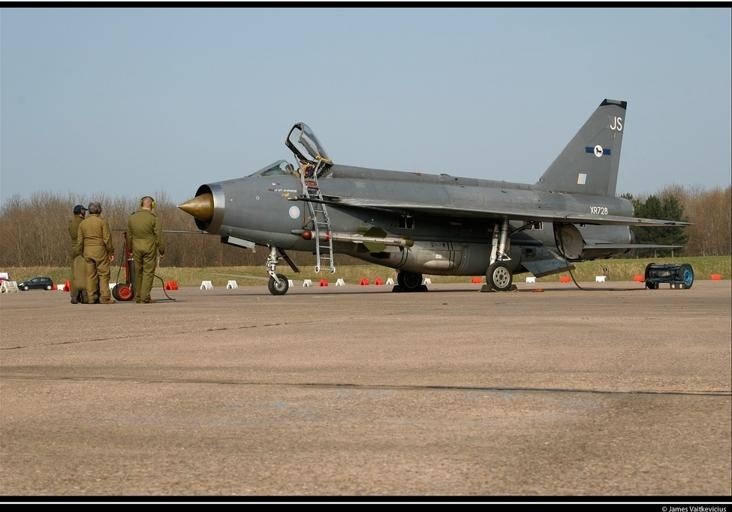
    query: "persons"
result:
[125,212,140,298]
[68,205,90,304]
[126,196,165,303]
[285,164,294,173]
[78,201,117,304]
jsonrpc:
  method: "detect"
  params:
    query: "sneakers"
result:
[101,300,115,303]
[71,297,97,304]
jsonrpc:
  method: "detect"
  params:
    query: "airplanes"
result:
[175,98,696,295]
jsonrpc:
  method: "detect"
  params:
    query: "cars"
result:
[18,276,53,291]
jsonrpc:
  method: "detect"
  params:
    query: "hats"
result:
[74,205,88,212]
